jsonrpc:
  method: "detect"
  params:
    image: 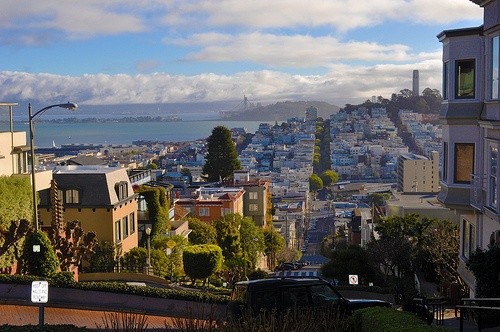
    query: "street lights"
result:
[28,101,79,232]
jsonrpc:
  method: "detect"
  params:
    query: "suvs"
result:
[232,275,394,317]
